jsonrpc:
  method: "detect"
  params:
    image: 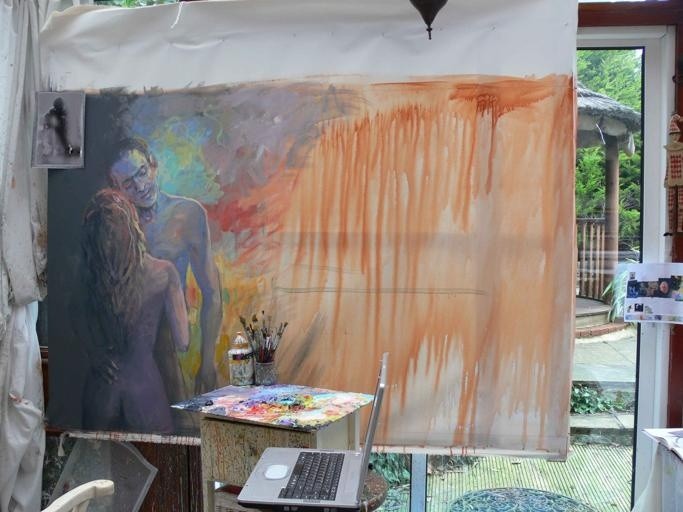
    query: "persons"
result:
[56,135,225,430]
[75,187,193,437]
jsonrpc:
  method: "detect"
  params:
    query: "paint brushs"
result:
[238,309,289,364]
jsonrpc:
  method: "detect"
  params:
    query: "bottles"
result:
[253,360,279,386]
[227,331,256,387]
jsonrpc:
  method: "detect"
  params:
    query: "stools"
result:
[360,470,388,512]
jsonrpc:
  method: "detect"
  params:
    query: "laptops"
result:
[236,351,389,512]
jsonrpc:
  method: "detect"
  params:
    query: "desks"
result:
[201,409,359,512]
